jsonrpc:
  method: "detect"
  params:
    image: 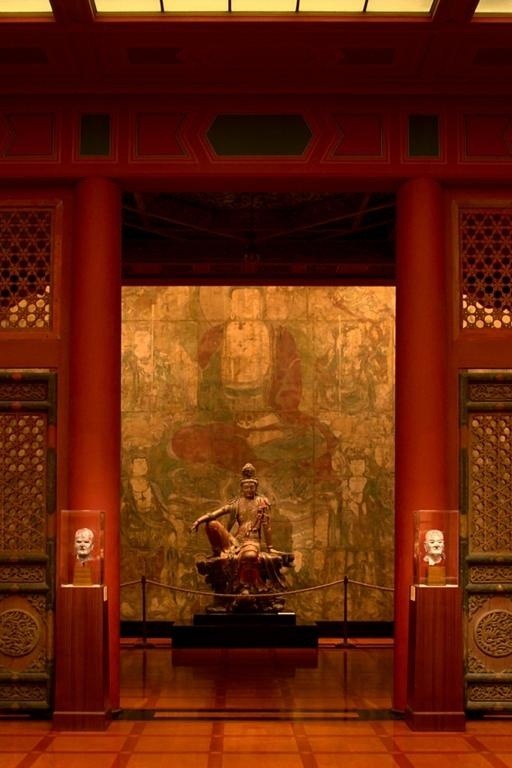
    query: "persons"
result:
[331,445,391,523]
[120,449,176,547]
[171,287,340,474]
[73,528,96,564]
[189,463,288,593]
[314,324,378,416]
[121,321,184,414]
[423,529,445,566]
